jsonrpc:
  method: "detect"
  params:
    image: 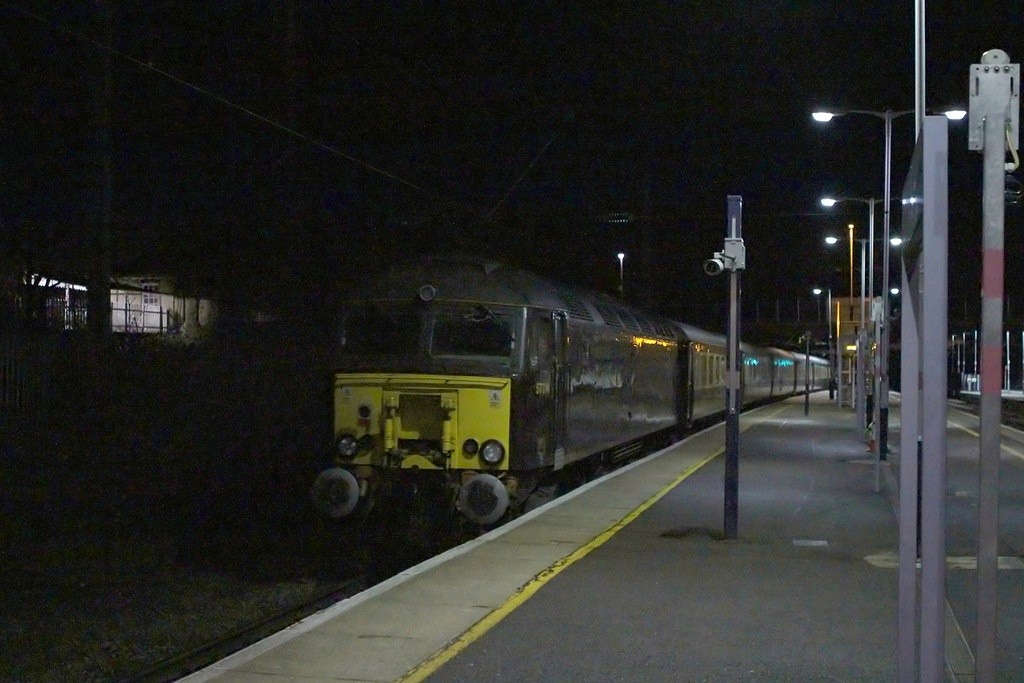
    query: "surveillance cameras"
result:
[703,258,723,276]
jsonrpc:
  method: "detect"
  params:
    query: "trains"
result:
[305,245,830,529]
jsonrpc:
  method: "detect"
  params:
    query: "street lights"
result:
[616,252,625,301]
[812,288,834,400]
[818,193,902,431]
[809,104,971,463]
[825,233,867,327]
